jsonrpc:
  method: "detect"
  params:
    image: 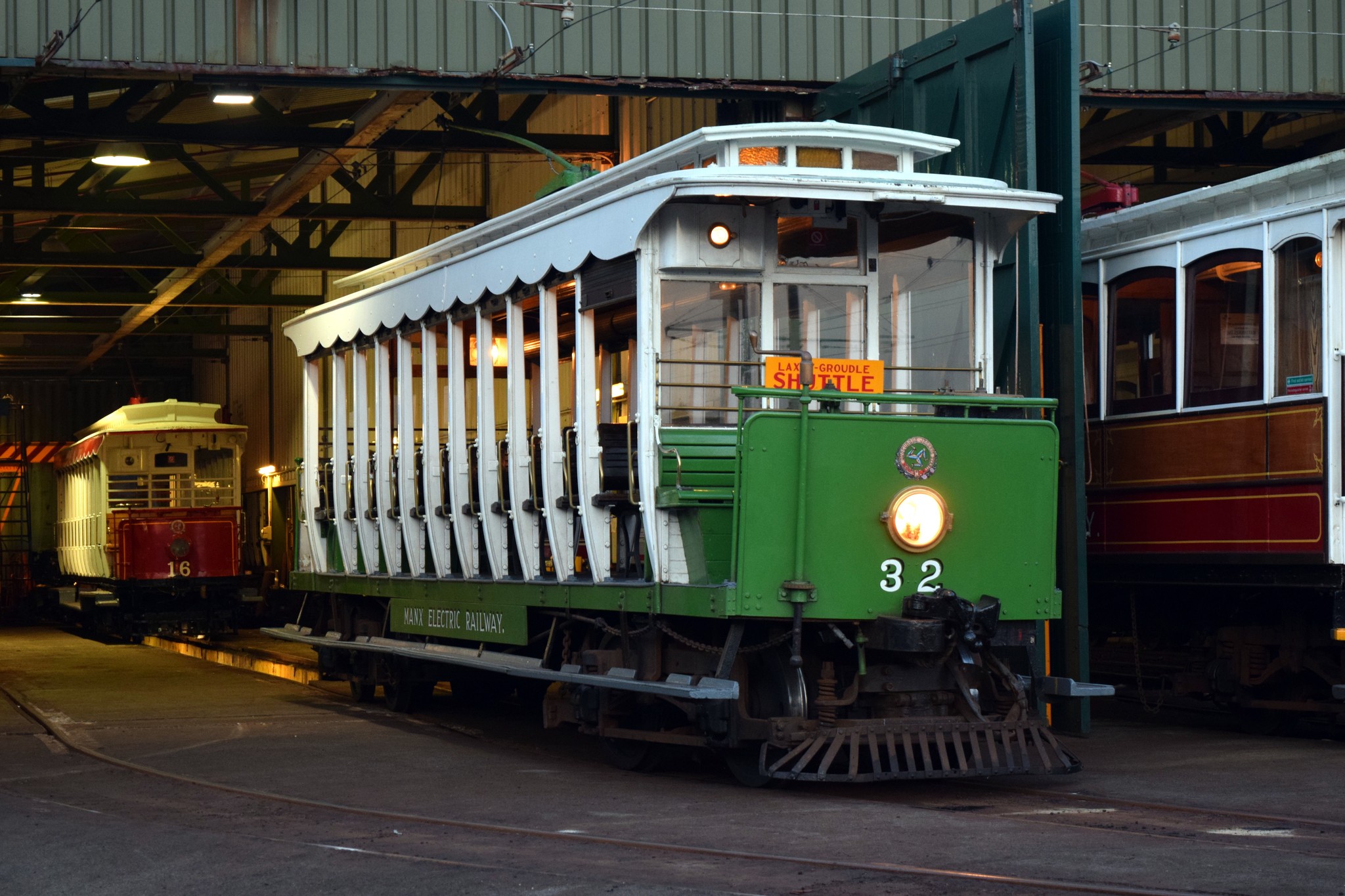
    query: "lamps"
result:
[20,287,44,298]
[92,142,150,166]
[207,87,259,105]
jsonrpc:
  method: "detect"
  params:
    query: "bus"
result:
[53,397,262,629]
[1080,149,1345,710]
[260,120,1116,784]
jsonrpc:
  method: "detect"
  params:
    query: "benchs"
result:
[314,423,744,583]
